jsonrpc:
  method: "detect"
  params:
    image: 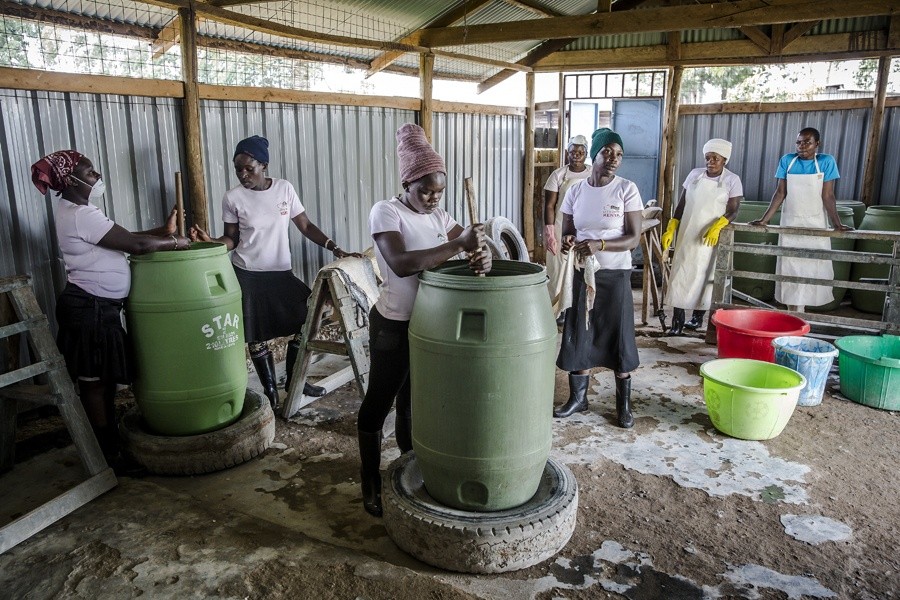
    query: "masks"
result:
[70,173,104,203]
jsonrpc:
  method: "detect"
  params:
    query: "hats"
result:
[590,128,624,161]
[703,138,733,165]
[566,135,588,153]
[396,123,447,182]
[235,135,269,164]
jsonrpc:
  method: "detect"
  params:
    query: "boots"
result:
[555,373,589,417]
[285,340,326,398]
[252,351,279,409]
[615,375,634,428]
[684,309,705,328]
[665,308,685,336]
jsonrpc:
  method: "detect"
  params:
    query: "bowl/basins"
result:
[834,334,899,413]
[630,263,657,286]
[700,358,806,440]
[668,247,675,258]
[711,308,810,363]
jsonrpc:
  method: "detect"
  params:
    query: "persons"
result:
[358,122,492,516]
[659,138,744,332]
[26,151,200,477]
[746,127,856,315]
[552,127,646,429]
[189,134,368,411]
[542,135,595,325]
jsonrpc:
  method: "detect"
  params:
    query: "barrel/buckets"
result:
[804,205,856,309]
[122,242,249,437]
[408,259,559,513]
[732,200,782,299]
[847,205,900,315]
[835,199,866,231]
[771,335,839,407]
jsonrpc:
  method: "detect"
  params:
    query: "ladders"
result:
[283,272,396,438]
[1,274,118,559]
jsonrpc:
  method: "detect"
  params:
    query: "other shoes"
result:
[104,454,147,478]
[363,494,384,518]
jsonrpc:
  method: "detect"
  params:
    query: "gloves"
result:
[661,218,679,250]
[545,225,557,256]
[703,216,729,247]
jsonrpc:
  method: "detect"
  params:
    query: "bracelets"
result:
[323,237,333,249]
[170,235,179,250]
[598,238,606,252]
